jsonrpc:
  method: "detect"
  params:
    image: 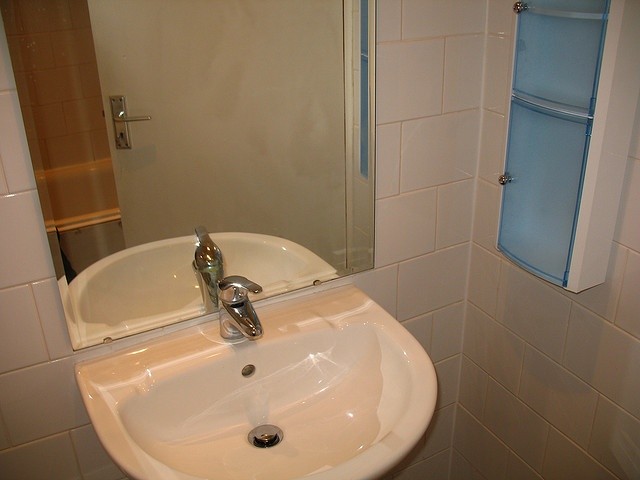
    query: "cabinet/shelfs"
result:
[494,0,639,293]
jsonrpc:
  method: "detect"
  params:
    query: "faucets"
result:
[192,226,224,313]
[218,275,263,340]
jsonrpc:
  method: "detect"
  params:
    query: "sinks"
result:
[74,283,438,480]
[68,232,338,355]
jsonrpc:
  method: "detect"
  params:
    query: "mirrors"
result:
[0,0,376,353]
